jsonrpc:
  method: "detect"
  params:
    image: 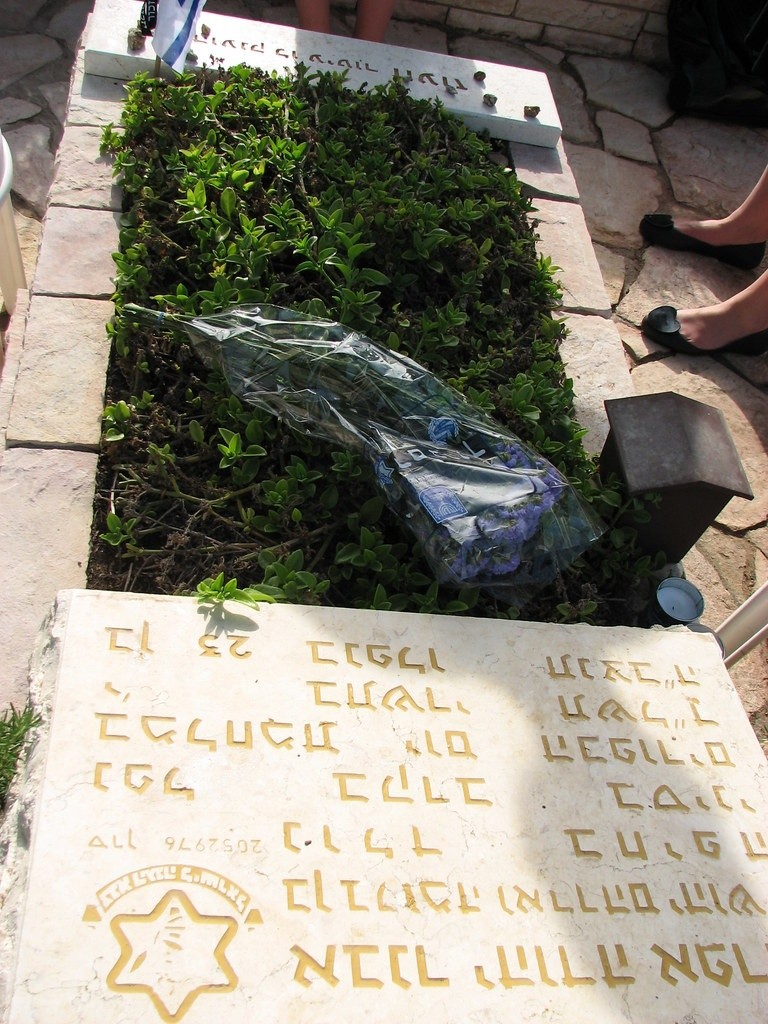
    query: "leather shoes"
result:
[640,213,768,271]
[642,306,767,356]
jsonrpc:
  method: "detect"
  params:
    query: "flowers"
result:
[123,299,572,586]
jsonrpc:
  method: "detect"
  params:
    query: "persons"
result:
[296,0,395,41]
[639,163,768,357]
